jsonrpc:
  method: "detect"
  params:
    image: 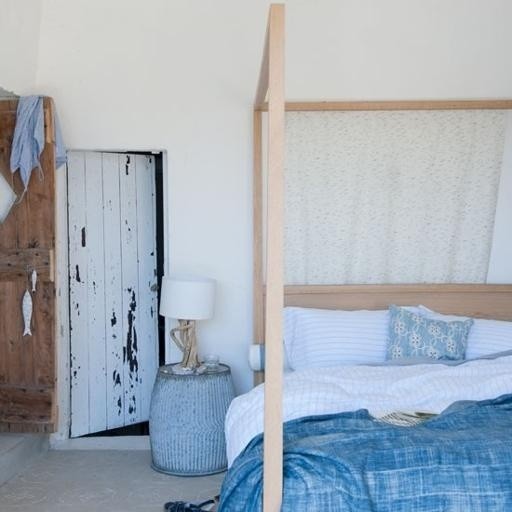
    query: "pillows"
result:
[244,303,512,367]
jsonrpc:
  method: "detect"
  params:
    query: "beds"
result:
[213,282,512,512]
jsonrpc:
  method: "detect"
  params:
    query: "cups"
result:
[203,353,219,372]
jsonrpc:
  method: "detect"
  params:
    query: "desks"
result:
[150,362,237,476]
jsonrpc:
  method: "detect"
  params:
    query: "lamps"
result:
[157,275,216,368]
